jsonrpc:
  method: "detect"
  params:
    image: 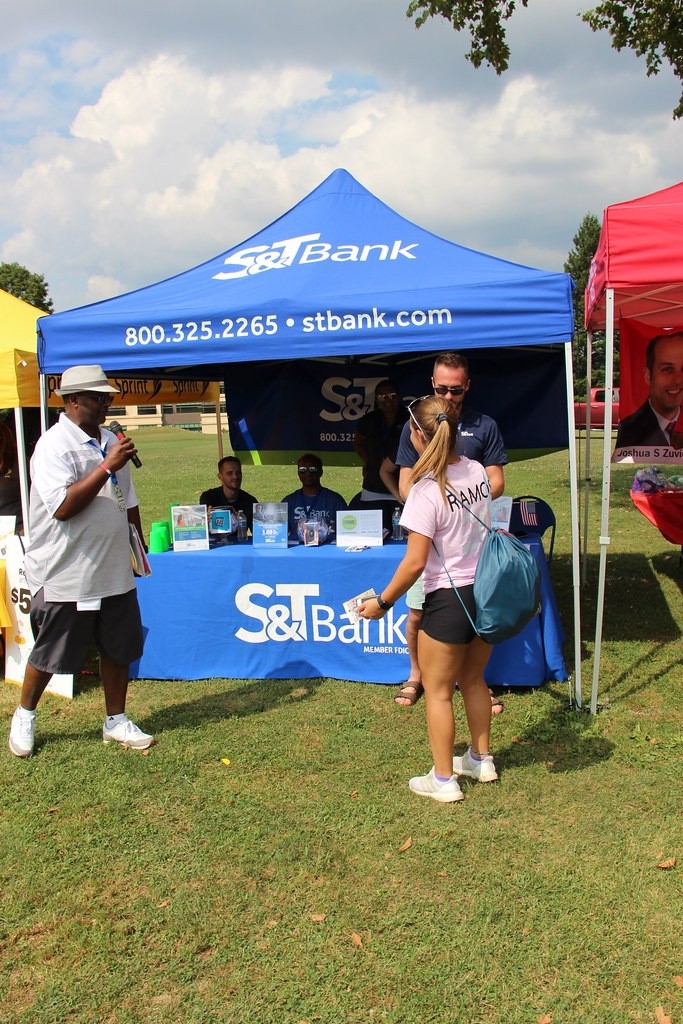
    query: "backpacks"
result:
[423,467,544,644]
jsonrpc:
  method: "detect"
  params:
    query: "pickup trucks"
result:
[573,388,621,429]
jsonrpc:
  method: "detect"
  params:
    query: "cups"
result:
[149,502,180,553]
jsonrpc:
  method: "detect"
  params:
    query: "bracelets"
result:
[99,463,113,477]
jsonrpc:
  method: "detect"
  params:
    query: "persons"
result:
[282,453,347,544]
[199,456,259,539]
[9,365,156,757]
[616,332,683,450]
[354,396,499,802]
[353,379,411,529]
[393,352,505,717]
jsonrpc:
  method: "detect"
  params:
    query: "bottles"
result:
[235,509,248,541]
[392,507,403,540]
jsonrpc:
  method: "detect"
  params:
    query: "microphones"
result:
[110,421,143,468]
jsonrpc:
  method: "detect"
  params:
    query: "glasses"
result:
[407,395,438,440]
[433,378,467,395]
[375,392,397,402]
[75,392,115,405]
[298,466,320,474]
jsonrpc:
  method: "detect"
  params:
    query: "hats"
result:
[54,365,121,397]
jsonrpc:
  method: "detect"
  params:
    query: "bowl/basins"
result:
[296,516,329,544]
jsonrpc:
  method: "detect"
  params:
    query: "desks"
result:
[128,533,572,686]
[629,488,683,568]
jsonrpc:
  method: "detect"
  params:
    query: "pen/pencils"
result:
[357,547,370,550]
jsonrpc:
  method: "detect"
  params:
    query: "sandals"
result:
[394,681,422,706]
[489,688,504,716]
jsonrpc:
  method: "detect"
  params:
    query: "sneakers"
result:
[408,765,465,802]
[453,746,498,782]
[9,706,37,757]
[102,719,156,749]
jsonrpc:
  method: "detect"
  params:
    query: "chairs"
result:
[508,496,556,570]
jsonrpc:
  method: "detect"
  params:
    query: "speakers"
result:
[0,406,66,516]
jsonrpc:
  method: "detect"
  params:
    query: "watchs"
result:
[378,593,394,611]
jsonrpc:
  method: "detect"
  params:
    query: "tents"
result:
[36,170,583,711]
[0,288,224,543]
[580,179,683,713]
